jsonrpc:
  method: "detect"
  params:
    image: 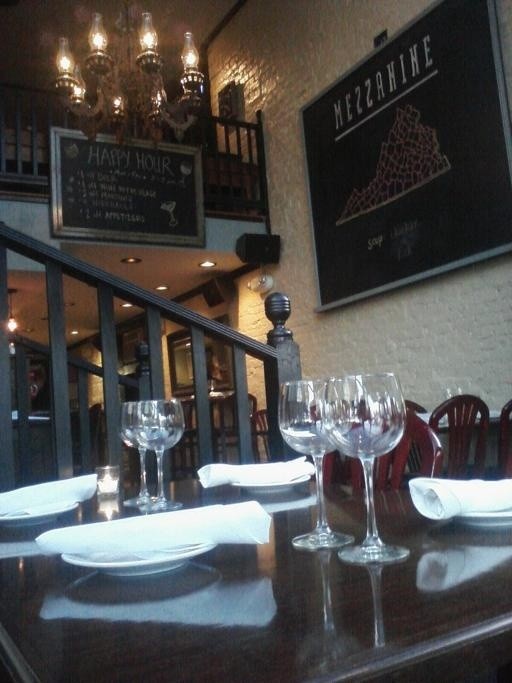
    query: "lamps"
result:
[54,0,208,148]
[8,287,19,332]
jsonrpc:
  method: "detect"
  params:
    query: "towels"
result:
[407,475,512,521]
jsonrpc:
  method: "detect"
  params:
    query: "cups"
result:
[276,375,354,553]
[439,375,473,400]
[93,464,121,499]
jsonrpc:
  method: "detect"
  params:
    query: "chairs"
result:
[88,392,278,477]
[316,394,512,489]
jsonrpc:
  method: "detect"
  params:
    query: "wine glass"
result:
[131,396,186,513]
[316,370,411,565]
[118,399,163,509]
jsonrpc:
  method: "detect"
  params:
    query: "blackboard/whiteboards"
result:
[299,0,512,313]
[50,126,206,247]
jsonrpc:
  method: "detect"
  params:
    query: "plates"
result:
[59,540,217,578]
[0,489,80,529]
[230,474,312,494]
[452,509,512,532]
